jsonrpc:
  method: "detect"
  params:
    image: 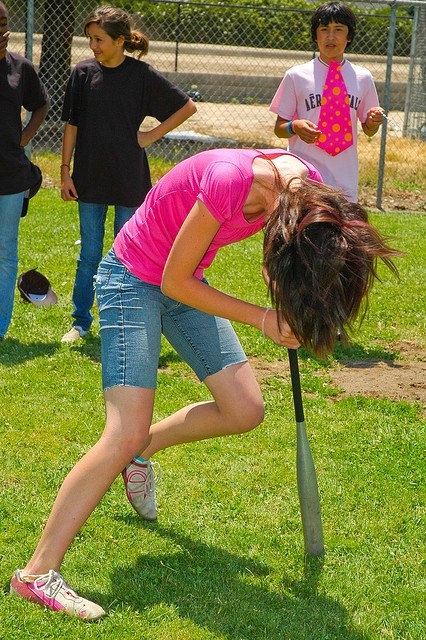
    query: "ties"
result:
[314,60,354,156]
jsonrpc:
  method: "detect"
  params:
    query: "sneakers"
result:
[62,328,90,344]
[121,457,162,521]
[9,568,106,623]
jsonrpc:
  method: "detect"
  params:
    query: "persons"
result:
[0,2,50,341]
[60,6,197,343]
[268,2,385,204]
[10,148,410,623]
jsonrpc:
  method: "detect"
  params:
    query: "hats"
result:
[21,165,42,217]
[17,267,57,307]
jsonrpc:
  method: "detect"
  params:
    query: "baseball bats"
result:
[287,348,325,558]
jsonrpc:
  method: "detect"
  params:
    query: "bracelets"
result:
[262,307,271,339]
[60,164,70,171]
[365,122,378,132]
[287,120,297,136]
